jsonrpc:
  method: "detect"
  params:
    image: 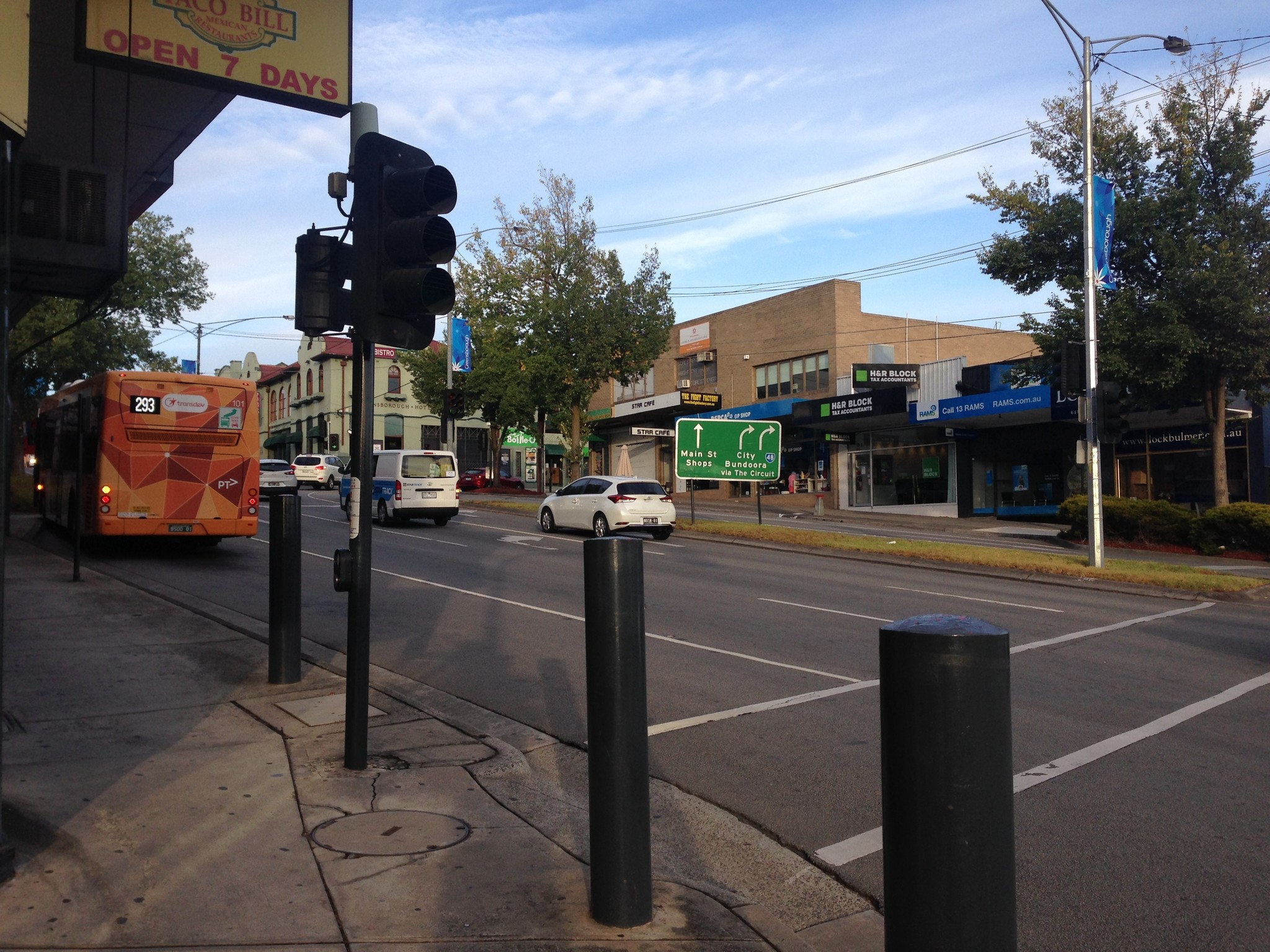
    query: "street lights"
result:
[143,308,295,374]
[1041,0,1194,568]
[444,227,529,458]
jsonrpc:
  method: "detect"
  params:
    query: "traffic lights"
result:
[442,387,465,418]
[353,131,458,349]
[292,230,355,337]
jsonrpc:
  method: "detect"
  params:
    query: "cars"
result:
[259,459,299,499]
[458,467,525,491]
[537,476,677,540]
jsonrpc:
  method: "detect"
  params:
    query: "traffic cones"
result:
[812,496,826,516]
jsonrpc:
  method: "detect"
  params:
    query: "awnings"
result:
[545,444,567,455]
[285,431,302,443]
[263,434,287,450]
[306,426,320,438]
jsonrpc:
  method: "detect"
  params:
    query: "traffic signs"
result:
[675,418,781,481]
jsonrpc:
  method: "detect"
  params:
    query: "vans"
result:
[338,449,460,526]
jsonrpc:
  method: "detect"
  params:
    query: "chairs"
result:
[662,482,672,496]
[1034,491,1047,506]
[1001,492,1015,507]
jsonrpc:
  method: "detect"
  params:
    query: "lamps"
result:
[393,359,398,363]
[971,451,975,460]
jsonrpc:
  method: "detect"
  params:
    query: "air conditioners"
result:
[697,352,713,363]
[677,379,690,389]
[0,149,128,299]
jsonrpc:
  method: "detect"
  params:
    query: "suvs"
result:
[289,454,344,490]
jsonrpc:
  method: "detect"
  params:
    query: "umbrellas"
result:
[615,445,635,477]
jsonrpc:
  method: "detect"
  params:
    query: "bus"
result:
[21,371,261,548]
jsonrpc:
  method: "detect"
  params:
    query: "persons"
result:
[788,471,797,493]
[1017,474,1025,488]
